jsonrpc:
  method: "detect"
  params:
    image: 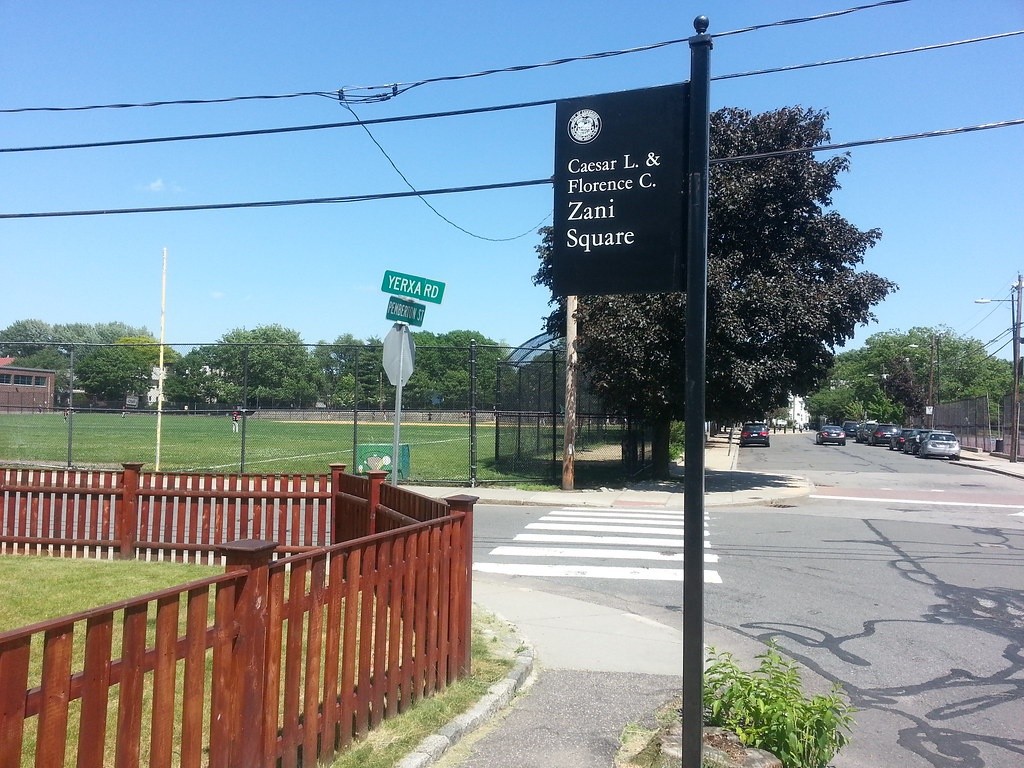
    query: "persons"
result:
[233,412,238,432]
[64,410,68,422]
[428,412,432,420]
[372,411,376,421]
[382,409,386,422]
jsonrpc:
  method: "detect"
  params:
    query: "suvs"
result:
[842,421,859,437]
[903,429,932,455]
[855,423,877,443]
[868,424,898,444]
[738,423,770,446]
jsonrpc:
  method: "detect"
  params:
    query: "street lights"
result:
[57,345,73,466]
[974,298,1022,463]
[240,346,248,473]
[909,344,934,429]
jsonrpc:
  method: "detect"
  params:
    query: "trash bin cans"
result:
[996,439,1003,452]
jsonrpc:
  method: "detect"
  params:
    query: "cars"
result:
[920,431,960,460]
[816,425,846,445]
[889,428,915,450]
